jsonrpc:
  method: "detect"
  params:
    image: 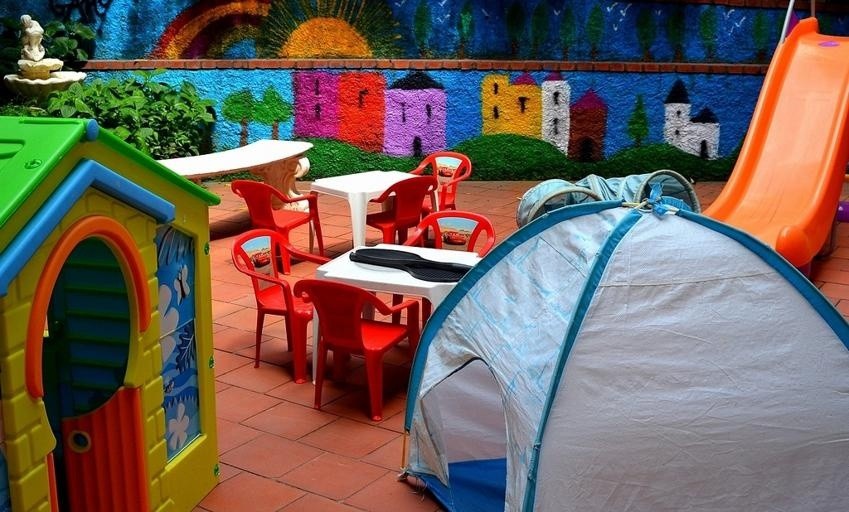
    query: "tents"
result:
[401,197,849,512]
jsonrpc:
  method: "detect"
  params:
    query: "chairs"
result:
[352,175,437,249]
[231,230,331,384]
[407,151,471,240]
[392,210,495,347]
[231,179,324,274]
[293,280,419,422]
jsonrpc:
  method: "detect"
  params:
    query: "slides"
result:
[700,16,849,268]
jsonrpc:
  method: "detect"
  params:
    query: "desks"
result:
[157,138,313,233]
[313,244,483,386]
[310,170,438,253]
